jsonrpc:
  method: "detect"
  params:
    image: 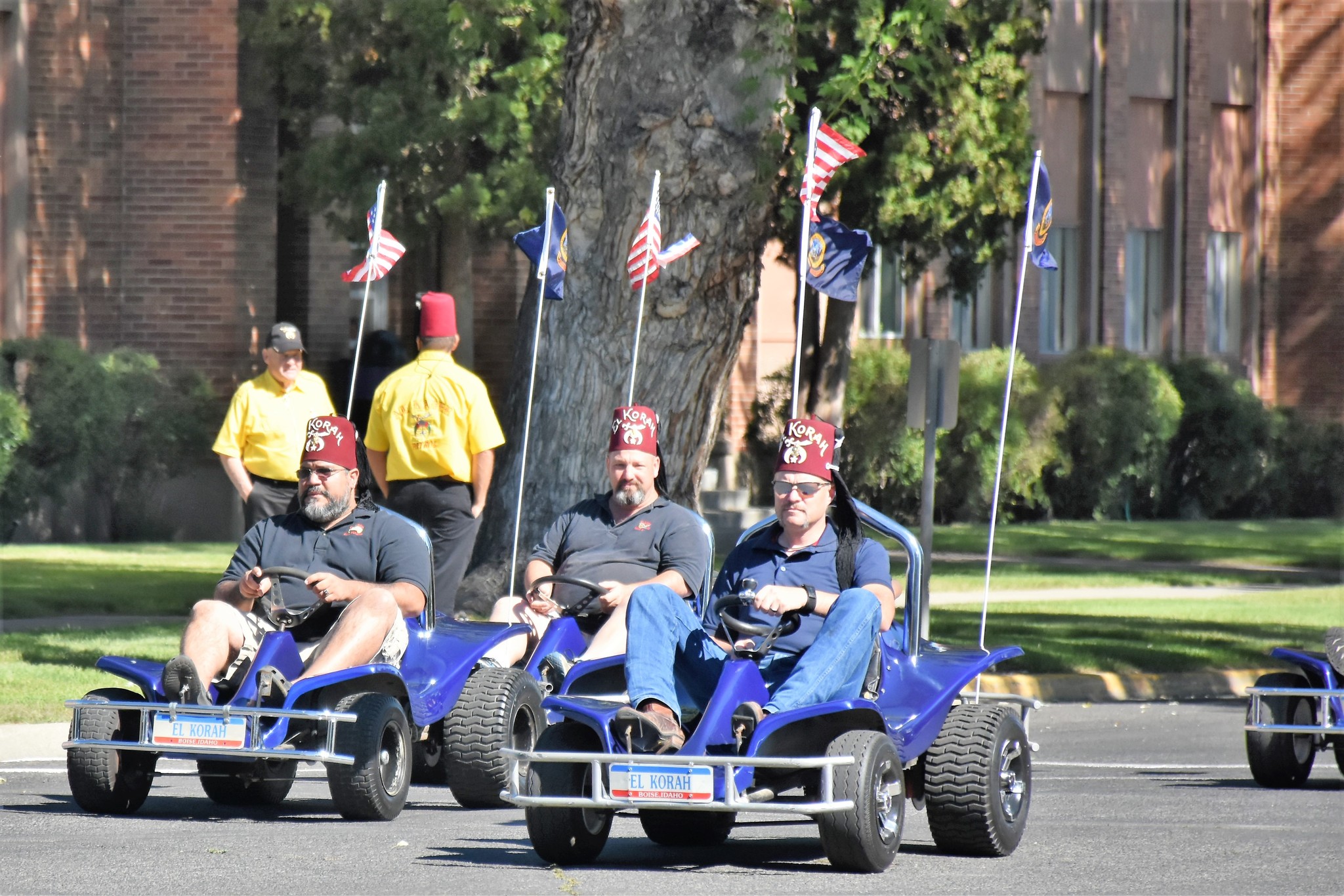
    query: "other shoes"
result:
[162,654,212,706]
[539,649,585,696]
[470,655,501,674]
[256,663,294,706]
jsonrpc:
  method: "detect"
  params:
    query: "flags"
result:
[627,170,700,290]
[1023,150,1059,272]
[513,187,568,300]
[800,107,873,302]
[341,179,406,282]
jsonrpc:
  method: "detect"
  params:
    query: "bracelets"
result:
[797,584,817,617]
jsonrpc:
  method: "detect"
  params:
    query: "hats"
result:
[299,416,357,471]
[266,321,309,357]
[419,291,457,337]
[609,406,658,457]
[774,418,835,482]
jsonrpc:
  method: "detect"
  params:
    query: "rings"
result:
[323,589,329,596]
[769,607,778,613]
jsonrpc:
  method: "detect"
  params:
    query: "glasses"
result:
[772,480,831,496]
[295,467,350,479]
[268,346,303,362]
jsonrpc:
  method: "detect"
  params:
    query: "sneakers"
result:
[732,699,769,748]
[615,707,685,755]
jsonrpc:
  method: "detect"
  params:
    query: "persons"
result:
[212,322,337,534]
[616,414,895,752]
[162,414,433,729]
[364,291,506,619]
[891,579,902,600]
[472,402,710,694]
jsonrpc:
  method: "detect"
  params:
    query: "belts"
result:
[251,474,299,488]
